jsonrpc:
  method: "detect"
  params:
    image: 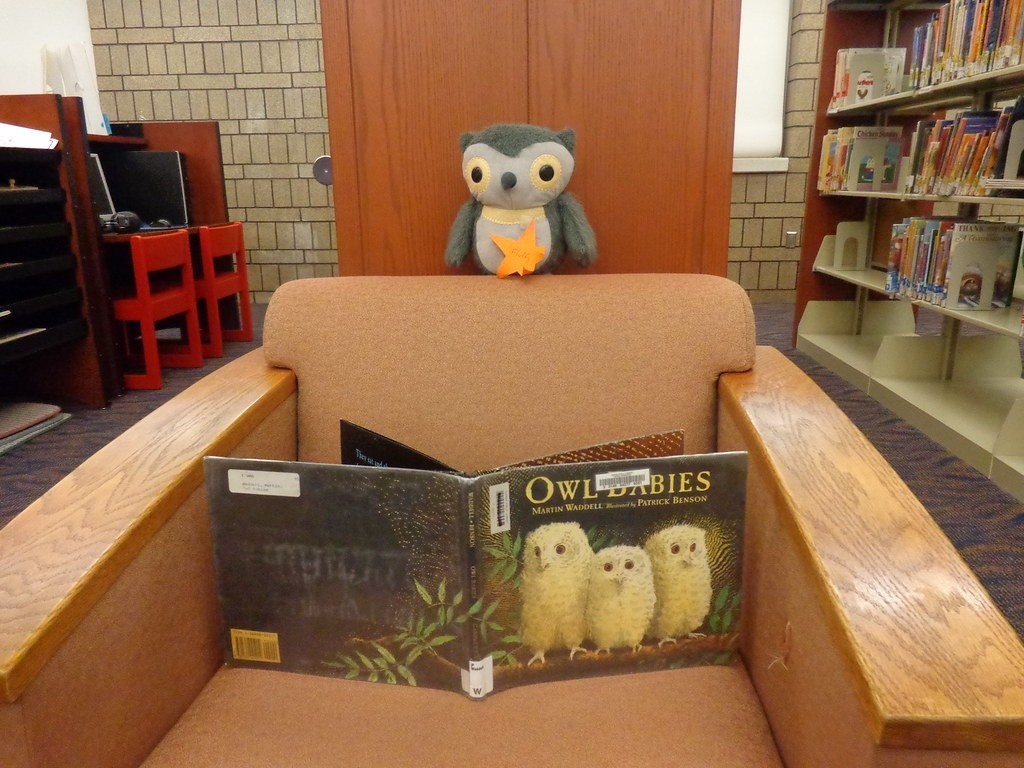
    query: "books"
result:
[202,418,747,703]
[816,0,1024,311]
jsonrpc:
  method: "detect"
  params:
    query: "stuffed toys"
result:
[443,124,596,278]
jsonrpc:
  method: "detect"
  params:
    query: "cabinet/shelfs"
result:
[0,92,252,408]
[793,0,1024,501]
[318,0,741,275]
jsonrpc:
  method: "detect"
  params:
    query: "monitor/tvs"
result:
[86,154,116,221]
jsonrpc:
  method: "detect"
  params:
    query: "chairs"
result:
[194,221,253,360]
[110,230,203,389]
[0,277,1024,768]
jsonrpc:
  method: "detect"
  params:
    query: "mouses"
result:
[149,219,172,227]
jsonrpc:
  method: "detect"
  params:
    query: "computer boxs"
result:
[98,150,192,225]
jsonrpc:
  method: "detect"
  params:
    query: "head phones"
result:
[101,211,142,232]
